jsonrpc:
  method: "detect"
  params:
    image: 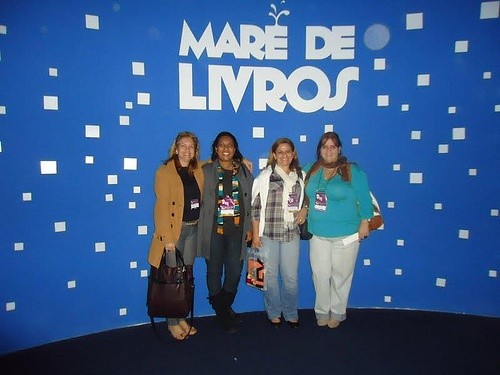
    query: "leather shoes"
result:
[271,318,299,332]
[317,318,340,328]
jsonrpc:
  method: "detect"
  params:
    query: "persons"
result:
[197,132,254,335]
[147,131,253,340]
[300,132,373,327]
[251,138,310,322]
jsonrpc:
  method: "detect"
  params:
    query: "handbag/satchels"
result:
[366,191,384,230]
[245,245,270,291]
[298,219,313,240]
[147,246,195,318]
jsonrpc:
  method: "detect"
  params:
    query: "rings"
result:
[300,219,302,222]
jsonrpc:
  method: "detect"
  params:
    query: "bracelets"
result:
[303,205,309,211]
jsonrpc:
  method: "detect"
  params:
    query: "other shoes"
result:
[168,320,197,340]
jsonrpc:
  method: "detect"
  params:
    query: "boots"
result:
[206,289,242,333]
